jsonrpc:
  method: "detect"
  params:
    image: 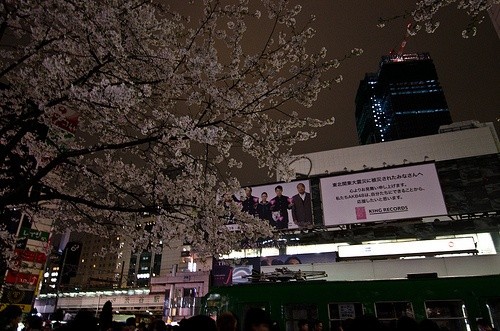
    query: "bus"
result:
[199,268,500,331]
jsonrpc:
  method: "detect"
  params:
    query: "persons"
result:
[256,192,274,229]
[285,257,302,265]
[0,303,500,331]
[239,187,260,218]
[291,183,313,228]
[268,186,292,230]
